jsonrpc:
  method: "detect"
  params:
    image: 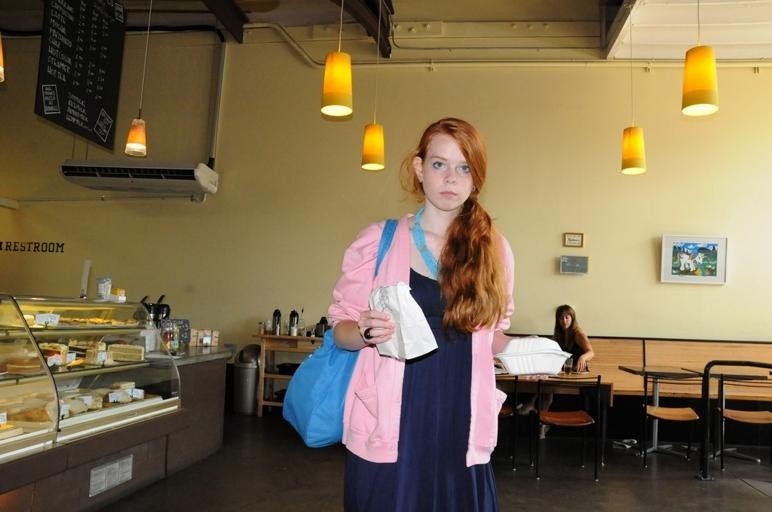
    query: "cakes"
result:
[0,382,146,440]
[22,315,34,326]
[57,318,138,328]
[0,340,144,374]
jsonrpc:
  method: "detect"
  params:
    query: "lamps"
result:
[321,1,354,117]
[681,1,718,119]
[621,12,646,176]
[362,0,386,172]
[123,1,153,156]
[0,38,6,82]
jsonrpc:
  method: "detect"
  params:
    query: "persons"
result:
[324,118,550,512]
[553,304,595,373]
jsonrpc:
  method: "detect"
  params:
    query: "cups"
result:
[319,317,328,337]
[97,276,111,299]
[564,358,573,376]
[258,322,265,337]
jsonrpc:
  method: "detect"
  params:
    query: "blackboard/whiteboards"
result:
[33,0,128,155]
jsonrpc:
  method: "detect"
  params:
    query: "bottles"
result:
[266,319,272,331]
[283,320,288,334]
[311,328,315,337]
[299,309,306,337]
[160,319,190,353]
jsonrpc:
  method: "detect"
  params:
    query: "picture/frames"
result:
[661,234,728,284]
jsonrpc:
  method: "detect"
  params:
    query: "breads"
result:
[110,288,125,295]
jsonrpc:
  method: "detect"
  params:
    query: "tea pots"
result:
[289,310,299,336]
[273,309,281,336]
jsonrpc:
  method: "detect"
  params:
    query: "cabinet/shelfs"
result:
[0,301,192,512]
[256,336,322,418]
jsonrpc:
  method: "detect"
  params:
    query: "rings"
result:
[364,328,373,340]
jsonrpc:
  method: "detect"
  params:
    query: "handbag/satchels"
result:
[282,330,360,448]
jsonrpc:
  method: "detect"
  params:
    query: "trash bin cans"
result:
[234,343,261,415]
